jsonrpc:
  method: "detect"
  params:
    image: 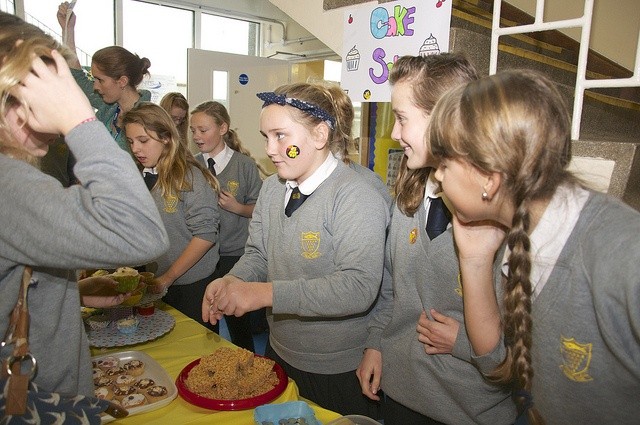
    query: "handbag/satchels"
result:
[1,266,128,425]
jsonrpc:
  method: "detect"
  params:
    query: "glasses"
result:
[171,116,186,122]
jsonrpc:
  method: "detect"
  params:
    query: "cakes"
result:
[104,368,125,375]
[98,356,116,365]
[91,360,99,367]
[97,377,108,386]
[142,271,155,283]
[88,316,112,330]
[112,375,136,386]
[91,269,108,277]
[112,399,123,405]
[118,317,141,334]
[93,387,112,397]
[120,291,143,307]
[92,367,104,375]
[121,394,146,403]
[113,266,141,292]
[80,307,97,316]
[148,387,168,395]
[134,379,155,388]
[124,359,144,371]
[136,304,157,316]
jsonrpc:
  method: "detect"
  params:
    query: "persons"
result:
[56,2,152,173]
[119,101,221,336]
[189,100,263,355]
[0,9,172,425]
[422,66,636,424]
[355,50,521,424]
[202,81,390,415]
[157,90,189,148]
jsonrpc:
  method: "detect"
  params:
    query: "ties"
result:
[142,172,156,190]
[285,187,309,216]
[207,158,215,175]
[425,198,451,240]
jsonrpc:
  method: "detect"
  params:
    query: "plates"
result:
[91,351,178,423]
[177,355,288,411]
